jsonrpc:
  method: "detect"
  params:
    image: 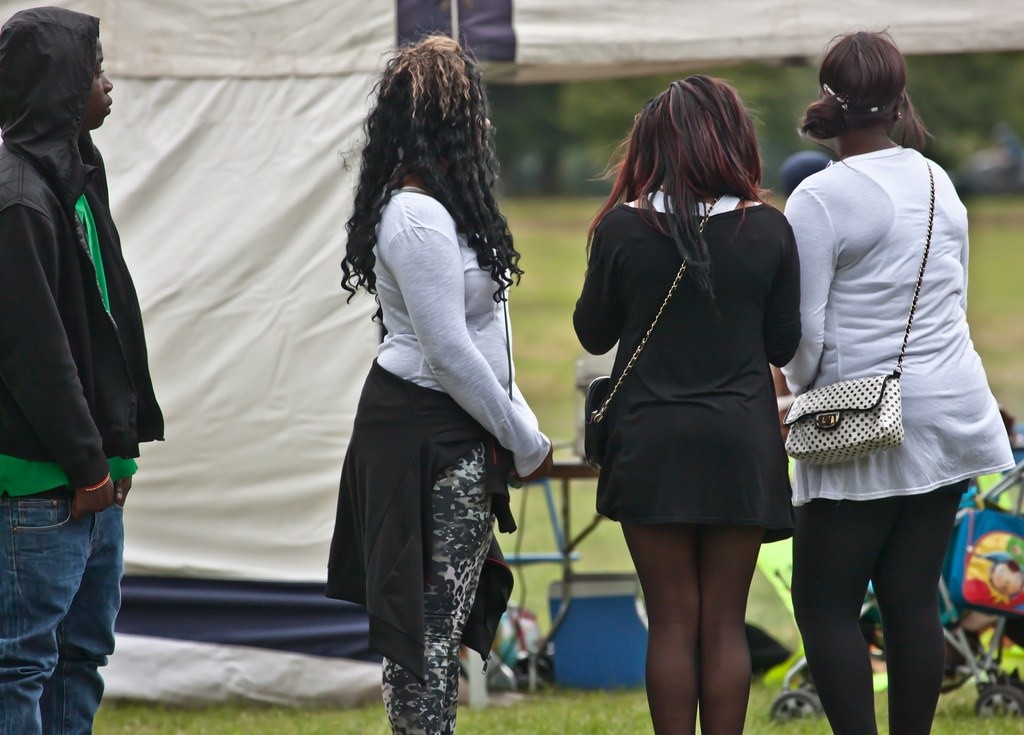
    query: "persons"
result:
[0,6,166,735]
[779,32,1016,735]
[572,77,801,735]
[326,36,554,735]
[773,149,835,444]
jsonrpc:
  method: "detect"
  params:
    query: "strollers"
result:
[766,455,1024,726]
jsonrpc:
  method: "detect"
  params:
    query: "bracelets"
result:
[78,472,110,492]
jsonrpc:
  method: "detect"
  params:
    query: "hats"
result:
[779,152,829,198]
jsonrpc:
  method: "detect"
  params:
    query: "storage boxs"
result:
[550,572,651,694]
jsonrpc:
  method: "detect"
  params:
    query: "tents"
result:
[0,0,1024,715]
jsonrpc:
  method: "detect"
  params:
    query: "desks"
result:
[482,456,608,694]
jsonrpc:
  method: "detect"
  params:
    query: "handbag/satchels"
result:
[584,377,613,472]
[782,371,905,466]
[948,507,1024,616]
